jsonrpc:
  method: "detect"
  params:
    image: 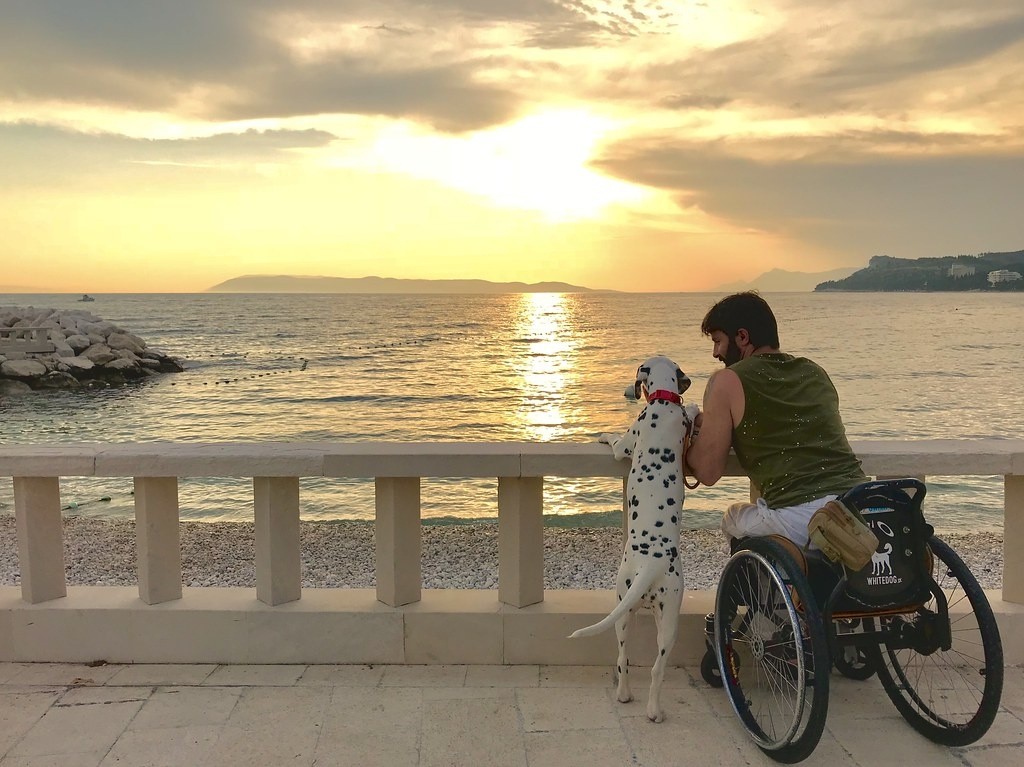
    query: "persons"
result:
[685,289,871,552]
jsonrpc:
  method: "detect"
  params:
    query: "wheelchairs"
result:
[700,478,1004,765]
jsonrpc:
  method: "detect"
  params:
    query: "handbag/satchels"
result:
[806,497,879,571]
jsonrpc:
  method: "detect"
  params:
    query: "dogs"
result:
[565,356,703,723]
